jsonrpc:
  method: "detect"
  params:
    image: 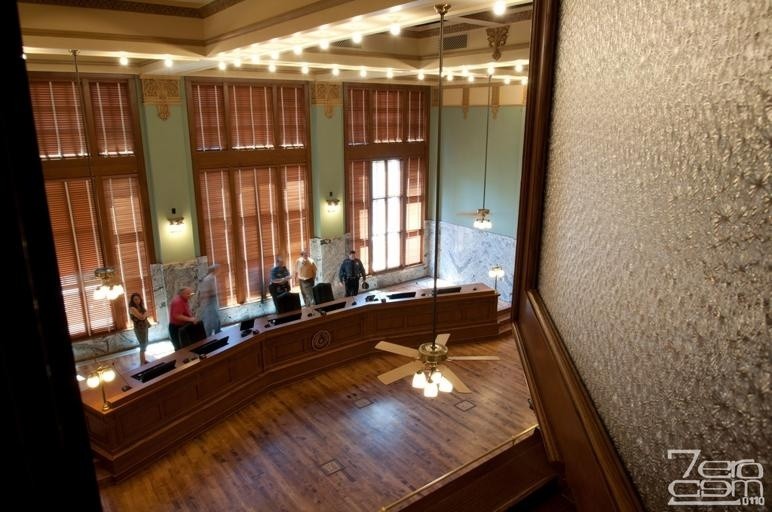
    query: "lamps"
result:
[473,218,492,229]
[93,285,123,301]
[168,217,186,236]
[86,366,116,411]
[412,369,453,398]
[489,264,504,289]
[326,198,341,213]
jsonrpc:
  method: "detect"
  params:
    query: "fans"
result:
[69,263,147,301]
[374,331,501,395]
[456,208,495,229]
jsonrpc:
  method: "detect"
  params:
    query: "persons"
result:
[194,263,222,338]
[168,287,198,352]
[268,255,292,312]
[338,248,369,297]
[128,292,153,365]
[292,250,305,284]
[299,250,317,306]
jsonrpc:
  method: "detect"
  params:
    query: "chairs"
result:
[277,292,301,313]
[313,283,335,304]
[177,321,206,347]
